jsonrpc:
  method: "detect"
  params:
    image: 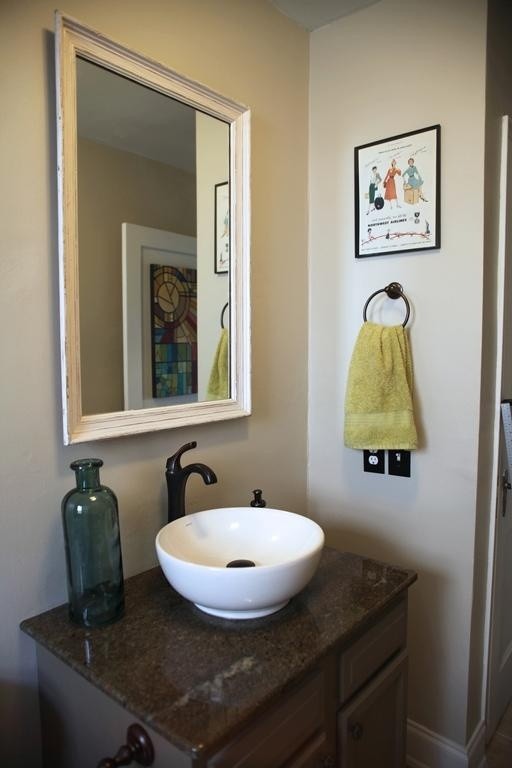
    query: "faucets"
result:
[164,442,219,520]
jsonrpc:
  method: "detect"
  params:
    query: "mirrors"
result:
[53,7,256,447]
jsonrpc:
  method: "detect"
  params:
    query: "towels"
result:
[343,323,419,452]
[204,328,230,400]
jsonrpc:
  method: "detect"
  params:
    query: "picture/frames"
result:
[351,123,442,259]
[213,181,229,275]
[120,222,196,410]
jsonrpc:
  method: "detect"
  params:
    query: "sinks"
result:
[155,506,325,621]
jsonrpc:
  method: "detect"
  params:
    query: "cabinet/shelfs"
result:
[204,598,409,767]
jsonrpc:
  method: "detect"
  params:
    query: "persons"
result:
[400,158,430,204]
[364,166,382,215]
[382,159,403,210]
